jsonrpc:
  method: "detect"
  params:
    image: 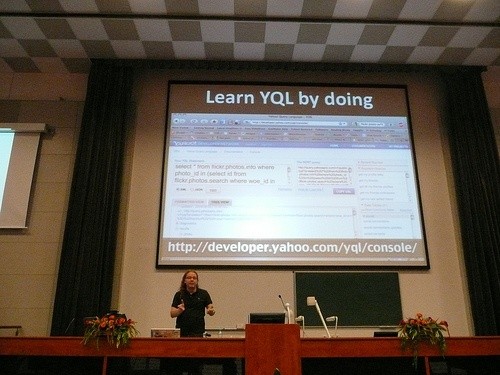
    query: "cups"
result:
[151,328,159,337]
[158,328,166,337]
[166,328,174,337]
[174,329,180,338]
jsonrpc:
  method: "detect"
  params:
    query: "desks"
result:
[0,335,500,375]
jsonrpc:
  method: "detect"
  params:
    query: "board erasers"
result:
[237,327,244,329]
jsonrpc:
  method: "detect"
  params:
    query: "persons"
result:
[170,270,215,337]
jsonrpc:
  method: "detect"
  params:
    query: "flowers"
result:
[80,310,141,352]
[395,313,451,368]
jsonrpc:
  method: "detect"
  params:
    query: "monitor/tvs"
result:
[151,328,180,338]
[374,332,398,337]
[250,313,285,324]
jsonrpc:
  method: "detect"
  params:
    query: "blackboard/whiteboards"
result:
[294,271,404,328]
[196,271,294,332]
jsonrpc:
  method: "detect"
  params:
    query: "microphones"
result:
[279,295,287,311]
